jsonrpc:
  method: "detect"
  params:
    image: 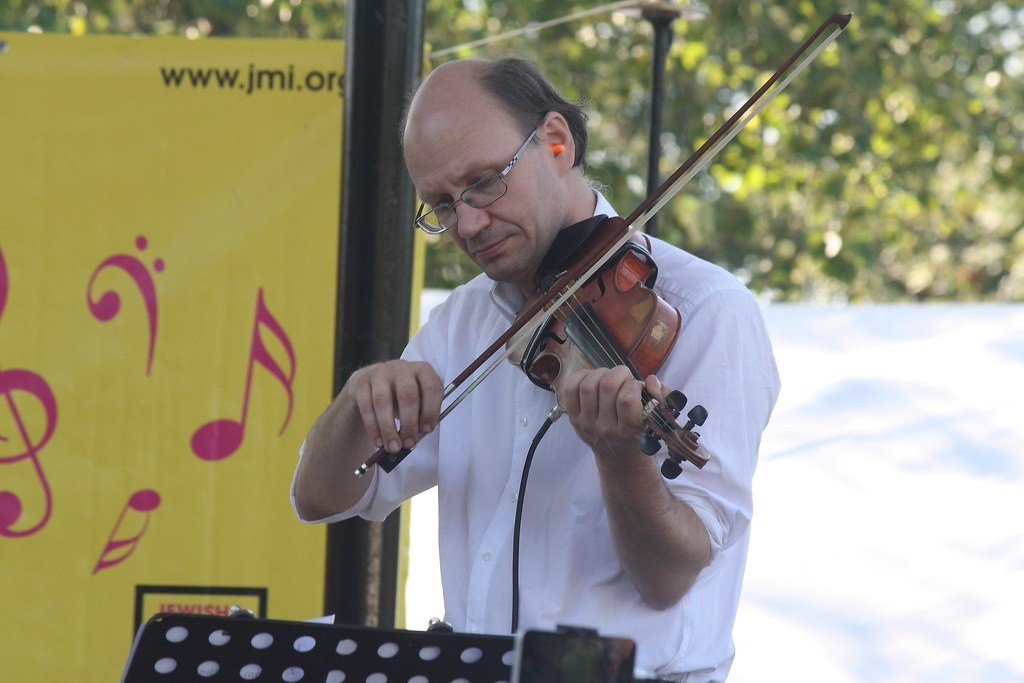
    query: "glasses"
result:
[412,125,541,236]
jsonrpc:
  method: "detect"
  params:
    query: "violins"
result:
[505,214,710,481]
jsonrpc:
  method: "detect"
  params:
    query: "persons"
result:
[288,51,783,683]
